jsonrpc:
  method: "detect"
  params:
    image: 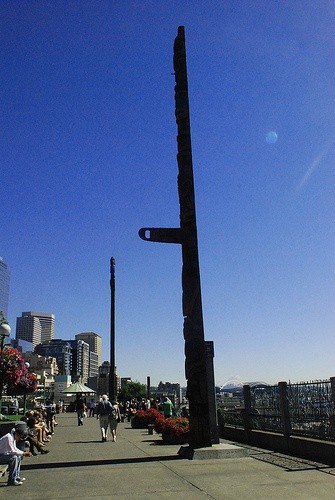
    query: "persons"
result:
[0,399,65,486]
[97,394,113,442]
[181,407,188,418]
[120,395,163,424]
[164,396,174,419]
[109,401,121,442]
[86,398,97,417]
[217,409,225,431]
[74,395,85,426]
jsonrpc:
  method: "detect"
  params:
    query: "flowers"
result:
[0,347,38,391]
[136,408,190,438]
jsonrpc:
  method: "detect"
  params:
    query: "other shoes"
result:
[32,453,37,456]
[41,422,58,446]
[113,435,116,442]
[17,478,25,482]
[7,481,22,486]
[102,437,107,442]
[41,450,49,454]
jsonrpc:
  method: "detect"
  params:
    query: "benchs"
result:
[0,463,9,479]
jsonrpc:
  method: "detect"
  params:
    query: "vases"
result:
[144,417,155,424]
[6,382,35,396]
[131,418,145,429]
[161,431,190,445]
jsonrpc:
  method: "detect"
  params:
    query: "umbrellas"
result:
[61,382,96,393]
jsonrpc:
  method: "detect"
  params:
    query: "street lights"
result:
[42,370,47,404]
[23,362,30,414]
[0,324,11,400]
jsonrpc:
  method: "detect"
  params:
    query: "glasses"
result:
[17,435,26,440]
